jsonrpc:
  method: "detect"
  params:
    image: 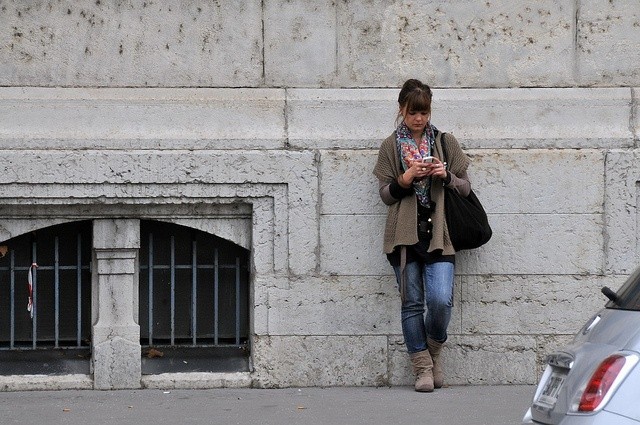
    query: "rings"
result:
[422,167,427,170]
[434,163,440,167]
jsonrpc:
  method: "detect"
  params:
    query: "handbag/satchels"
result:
[440,132,493,252]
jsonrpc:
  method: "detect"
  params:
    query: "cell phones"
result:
[423,156,432,164]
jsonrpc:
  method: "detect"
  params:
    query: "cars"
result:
[522,268,640,425]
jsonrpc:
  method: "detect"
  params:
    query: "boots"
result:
[409,349,434,392]
[426,337,444,389]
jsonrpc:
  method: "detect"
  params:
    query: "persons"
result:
[373,77,471,392]
